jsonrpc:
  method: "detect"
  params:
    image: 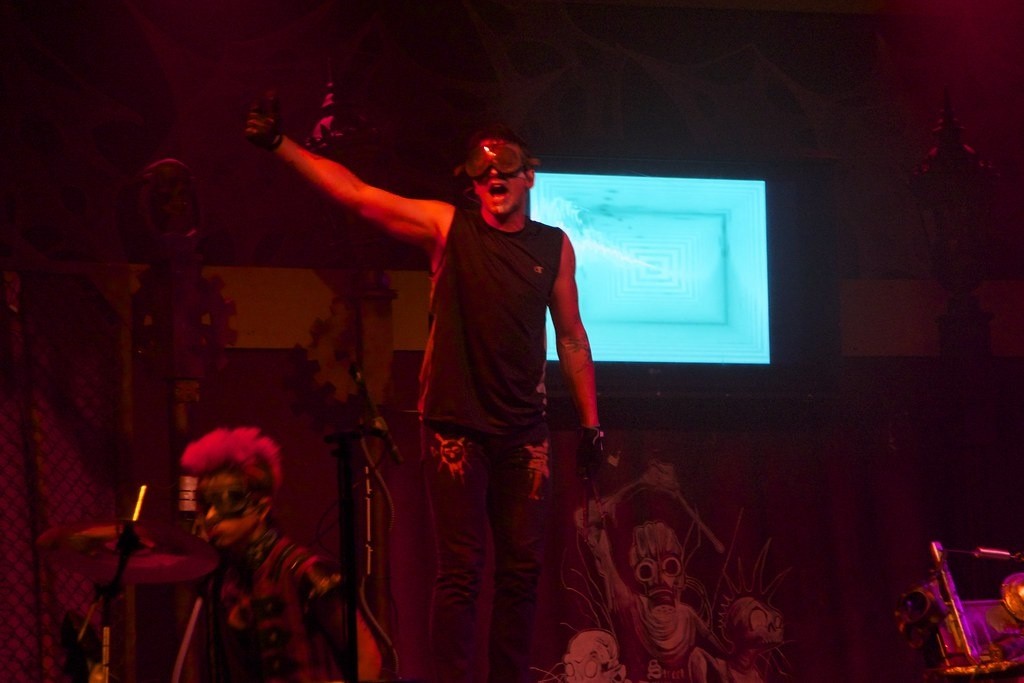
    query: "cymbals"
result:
[35,517,221,586]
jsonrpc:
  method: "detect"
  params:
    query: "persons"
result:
[242,96,605,683]
[175,424,384,683]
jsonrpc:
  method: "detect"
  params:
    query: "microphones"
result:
[375,413,406,465]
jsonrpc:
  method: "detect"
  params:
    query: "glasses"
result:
[466,142,527,179]
[193,482,260,519]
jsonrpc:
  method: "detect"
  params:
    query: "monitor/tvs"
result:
[524,155,800,385]
